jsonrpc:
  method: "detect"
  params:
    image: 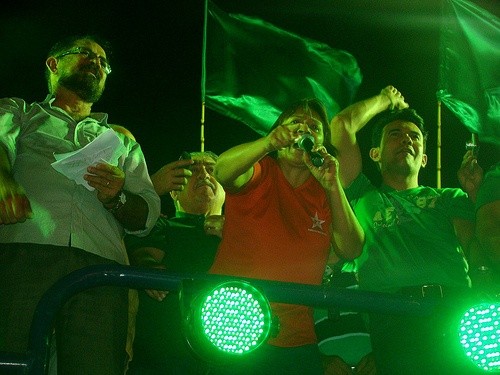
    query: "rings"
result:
[106,180,112,188]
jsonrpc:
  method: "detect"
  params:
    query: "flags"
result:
[435,0,500,149]
[200,0,364,161]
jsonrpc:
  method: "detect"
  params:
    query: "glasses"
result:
[56,47,112,75]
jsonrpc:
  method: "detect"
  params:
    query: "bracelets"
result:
[383,93,394,110]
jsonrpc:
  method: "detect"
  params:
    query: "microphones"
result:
[298,134,325,167]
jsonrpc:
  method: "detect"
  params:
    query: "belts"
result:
[397,284,462,301]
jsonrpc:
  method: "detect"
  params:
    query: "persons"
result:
[131,152,225,375]
[205,98,366,375]
[314,86,500,375]
[0,30,161,375]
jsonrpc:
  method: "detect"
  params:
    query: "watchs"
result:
[108,191,127,212]
